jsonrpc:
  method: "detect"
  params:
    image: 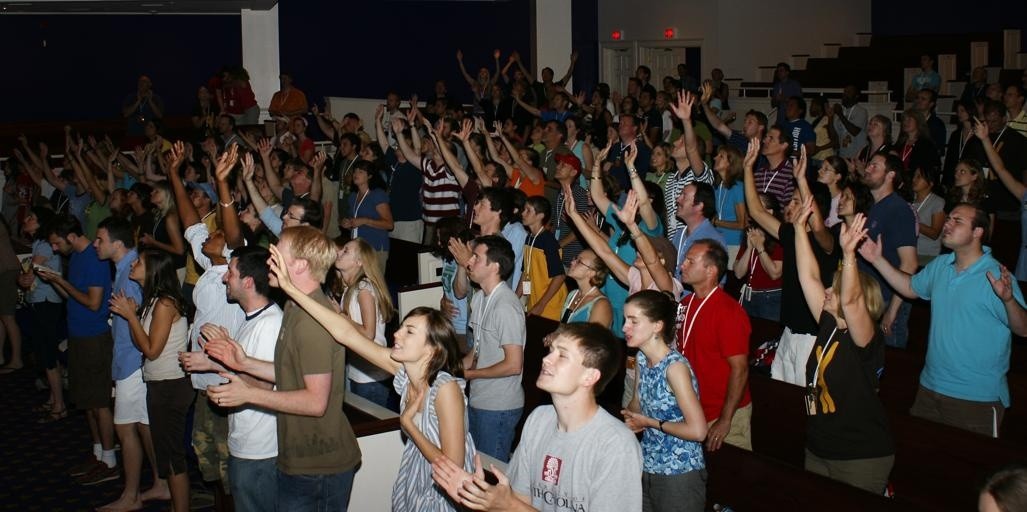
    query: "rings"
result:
[216,396,221,406]
[712,435,719,441]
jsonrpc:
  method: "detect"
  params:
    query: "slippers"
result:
[0,366,24,374]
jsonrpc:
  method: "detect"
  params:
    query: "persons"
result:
[2,48,1027,512]
[621,293,716,512]
[668,238,752,454]
[854,197,1027,440]
[262,242,477,509]
[201,225,363,512]
[432,320,646,512]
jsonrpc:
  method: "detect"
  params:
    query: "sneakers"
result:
[70,455,102,476]
[78,462,120,485]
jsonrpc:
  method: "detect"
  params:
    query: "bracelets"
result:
[658,418,667,432]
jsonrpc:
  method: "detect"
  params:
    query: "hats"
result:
[555,153,581,172]
[189,181,219,205]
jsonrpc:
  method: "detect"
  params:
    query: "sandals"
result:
[38,408,67,424]
[31,403,54,414]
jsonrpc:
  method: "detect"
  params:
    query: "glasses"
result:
[572,256,598,272]
[284,210,305,222]
